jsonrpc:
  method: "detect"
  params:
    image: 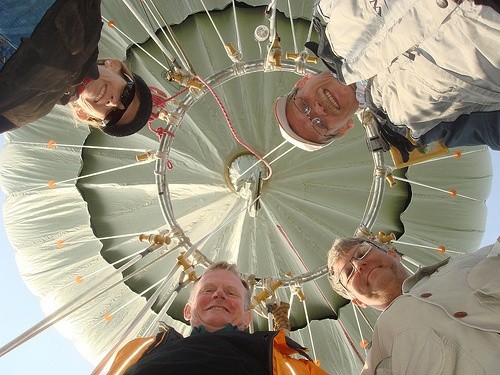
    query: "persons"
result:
[105,260,328,375]
[274,1,500,154]
[1,0,152,138]
[326,235,500,375]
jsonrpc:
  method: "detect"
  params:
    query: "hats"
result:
[98,73,152,138]
[275,90,334,152]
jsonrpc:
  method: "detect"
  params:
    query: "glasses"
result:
[90,72,135,128]
[336,239,388,293]
[292,89,338,138]
